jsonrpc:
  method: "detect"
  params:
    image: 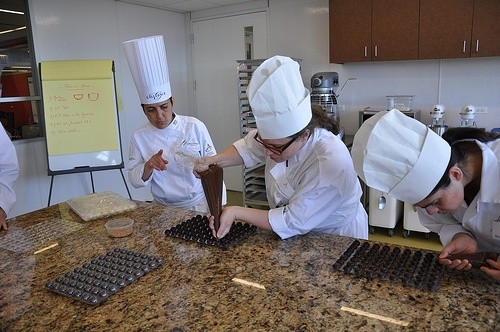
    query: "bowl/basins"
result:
[386,96,415,111]
[427,124,447,137]
[104,218,134,237]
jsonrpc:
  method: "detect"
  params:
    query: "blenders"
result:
[459,104,478,128]
[309,72,345,141]
[427,105,448,137]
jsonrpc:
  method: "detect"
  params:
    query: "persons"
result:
[0,123,20,232]
[128,86,227,212]
[192,56,370,240]
[350,109,500,281]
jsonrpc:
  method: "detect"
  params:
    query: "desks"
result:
[0,191,500,332]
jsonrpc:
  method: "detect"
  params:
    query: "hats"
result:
[122,34,173,105]
[246,56,313,140]
[351,109,451,205]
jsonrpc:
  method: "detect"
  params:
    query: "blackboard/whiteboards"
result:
[37,58,125,177]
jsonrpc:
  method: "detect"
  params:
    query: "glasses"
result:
[253,129,305,156]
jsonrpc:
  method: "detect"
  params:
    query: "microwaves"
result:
[359,107,421,128]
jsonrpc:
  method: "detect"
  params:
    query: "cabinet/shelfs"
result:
[329,0,421,64]
[236,57,305,210]
[418,0,500,60]
